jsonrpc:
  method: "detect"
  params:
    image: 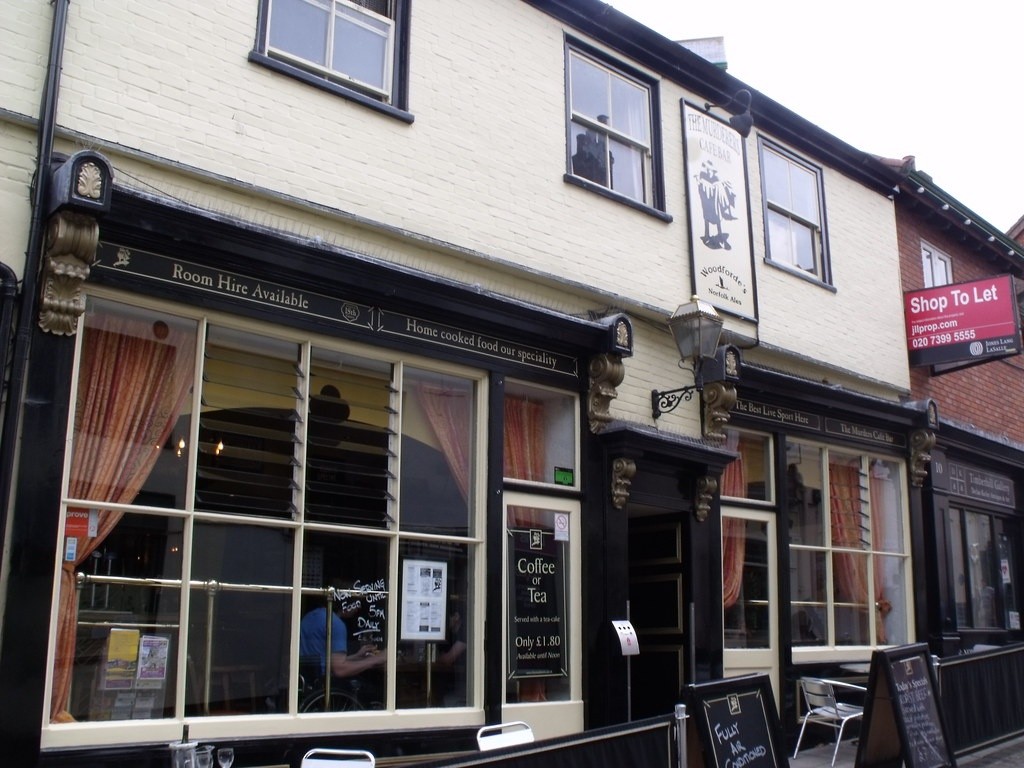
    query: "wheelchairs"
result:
[276,653,381,714]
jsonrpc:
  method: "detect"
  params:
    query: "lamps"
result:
[705,89,753,138]
[477,720,534,752]
[651,295,724,419]
[301,748,376,768]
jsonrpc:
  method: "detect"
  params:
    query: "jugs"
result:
[183,745,215,768]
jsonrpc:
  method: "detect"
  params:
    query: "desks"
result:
[374,653,443,708]
[841,663,871,746]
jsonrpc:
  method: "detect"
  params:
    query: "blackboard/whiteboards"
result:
[682,672,790,768]
[855,641,958,768]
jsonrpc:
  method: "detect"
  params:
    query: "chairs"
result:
[792,680,864,766]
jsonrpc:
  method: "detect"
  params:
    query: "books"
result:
[99,629,169,718]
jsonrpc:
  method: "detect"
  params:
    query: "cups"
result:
[217,748,233,768]
[416,643,426,663]
[168,740,198,768]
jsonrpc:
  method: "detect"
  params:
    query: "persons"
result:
[438,605,468,677]
[792,611,813,639]
[301,598,386,704]
